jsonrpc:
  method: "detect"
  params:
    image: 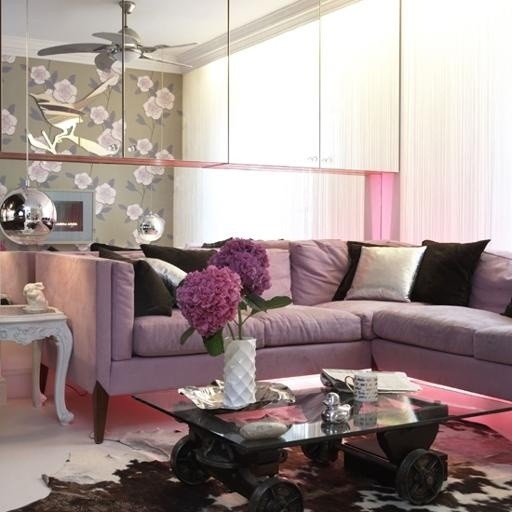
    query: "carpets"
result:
[6,413,512,512]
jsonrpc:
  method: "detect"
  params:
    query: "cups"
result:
[344,371,378,402]
[347,402,378,429]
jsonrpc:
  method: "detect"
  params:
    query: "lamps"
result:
[112,50,141,62]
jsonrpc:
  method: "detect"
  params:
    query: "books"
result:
[259,367,373,395]
[321,365,422,393]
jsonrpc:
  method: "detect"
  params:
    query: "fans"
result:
[37,0,197,69]
